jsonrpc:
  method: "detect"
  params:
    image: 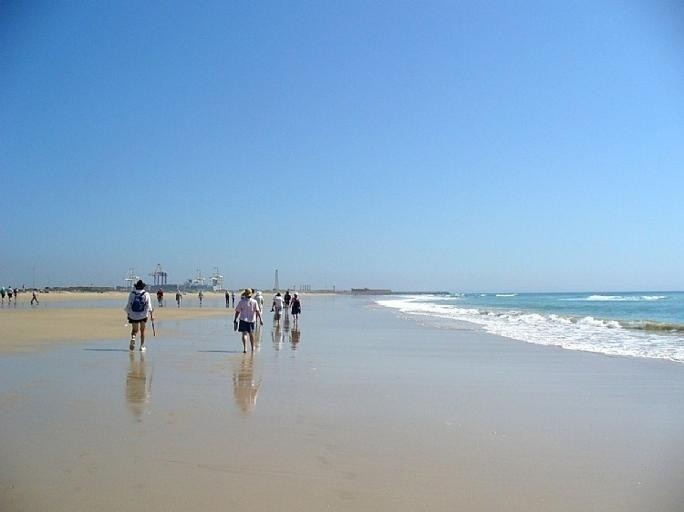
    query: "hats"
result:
[133,280,146,287]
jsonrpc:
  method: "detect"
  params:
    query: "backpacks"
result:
[131,290,146,312]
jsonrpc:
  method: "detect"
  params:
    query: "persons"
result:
[223,287,265,351]
[196,290,206,302]
[270,288,302,324]
[173,289,185,306]
[157,288,165,304]
[1,285,45,306]
[231,350,265,415]
[124,281,154,352]
[273,321,306,353]
[125,353,153,423]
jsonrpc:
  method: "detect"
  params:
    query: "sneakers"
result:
[130,339,135,350]
[140,346,146,350]
